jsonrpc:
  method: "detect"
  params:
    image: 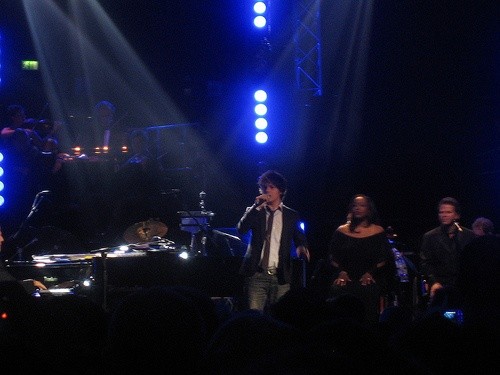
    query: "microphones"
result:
[452,219,463,232]
[39,190,50,195]
[255,199,265,208]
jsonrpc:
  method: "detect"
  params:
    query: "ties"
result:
[260,206,279,273]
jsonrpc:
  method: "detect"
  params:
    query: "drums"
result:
[177,208,215,234]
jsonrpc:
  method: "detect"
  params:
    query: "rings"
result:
[367,277,371,281]
[340,278,345,281]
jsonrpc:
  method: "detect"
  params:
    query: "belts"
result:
[267,269,277,275]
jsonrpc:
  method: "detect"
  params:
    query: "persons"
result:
[418,197,497,310]
[0,260,500,375]
[326,193,392,322]
[0,100,155,218]
[236,170,310,314]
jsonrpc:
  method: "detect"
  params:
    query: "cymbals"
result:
[122,218,168,244]
